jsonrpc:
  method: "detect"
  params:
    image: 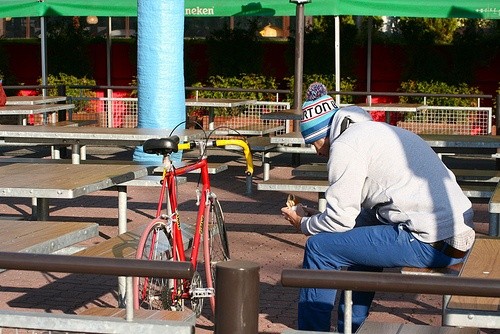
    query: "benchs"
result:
[0,122,500,334]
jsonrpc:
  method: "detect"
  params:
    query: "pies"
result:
[287,194,298,207]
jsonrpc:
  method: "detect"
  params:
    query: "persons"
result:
[281,81,476,334]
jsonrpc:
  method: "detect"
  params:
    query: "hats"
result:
[299,82,340,144]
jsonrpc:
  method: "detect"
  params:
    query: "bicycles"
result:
[131,119,255,322]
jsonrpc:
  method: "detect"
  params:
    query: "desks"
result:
[270,132,500,202]
[0,125,215,164]
[0,221,99,280]
[257,178,499,203]
[0,163,148,221]
[0,103,76,147]
[5,96,73,124]
[186,98,257,150]
[362,103,426,125]
[261,109,304,166]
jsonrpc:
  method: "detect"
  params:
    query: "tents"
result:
[0,0,500,128]
